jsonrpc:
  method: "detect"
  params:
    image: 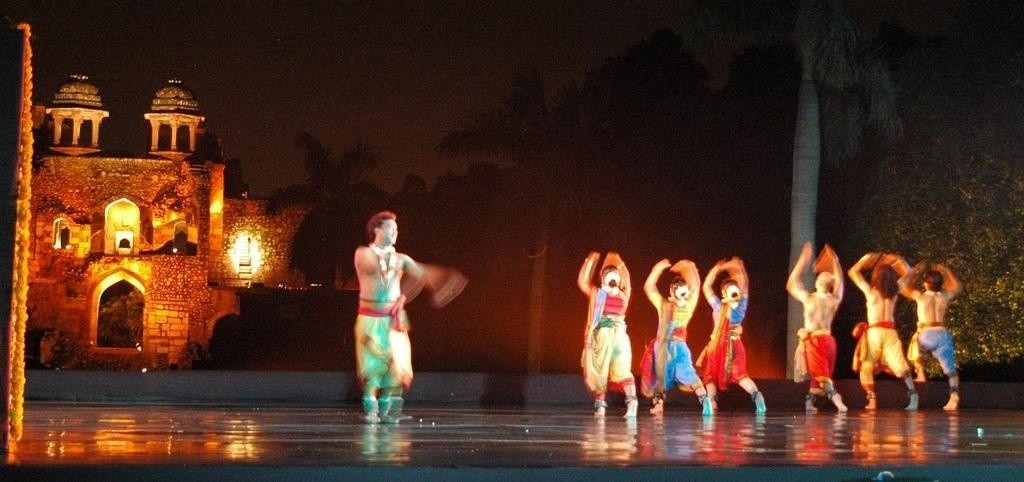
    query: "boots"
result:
[361,397,402,426]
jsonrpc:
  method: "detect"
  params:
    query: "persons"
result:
[577,251,639,417]
[899,261,963,411]
[696,256,767,413]
[353,212,468,423]
[847,252,919,410]
[786,241,848,413]
[639,259,713,416]
